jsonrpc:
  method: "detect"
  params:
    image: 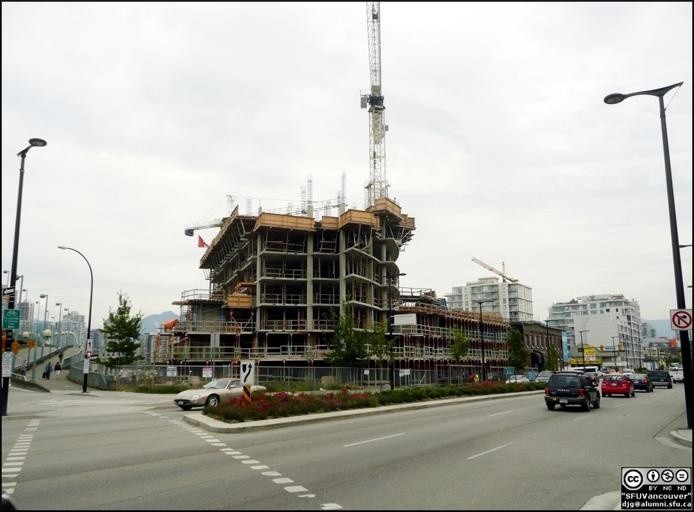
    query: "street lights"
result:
[574,328,588,369]
[610,332,620,369]
[545,315,562,381]
[383,272,409,392]
[3,269,80,382]
[3,134,45,416]
[473,298,497,381]
[604,81,692,433]
[58,243,95,398]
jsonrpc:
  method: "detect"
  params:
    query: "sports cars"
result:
[172,378,265,411]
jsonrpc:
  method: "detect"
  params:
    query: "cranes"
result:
[174,187,350,239]
[358,3,393,201]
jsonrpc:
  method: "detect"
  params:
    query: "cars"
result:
[506,362,684,412]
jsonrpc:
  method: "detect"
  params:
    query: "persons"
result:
[58,350,64,363]
[53,361,62,380]
[20,359,28,376]
[44,360,53,380]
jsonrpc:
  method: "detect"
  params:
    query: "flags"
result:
[198,235,206,247]
